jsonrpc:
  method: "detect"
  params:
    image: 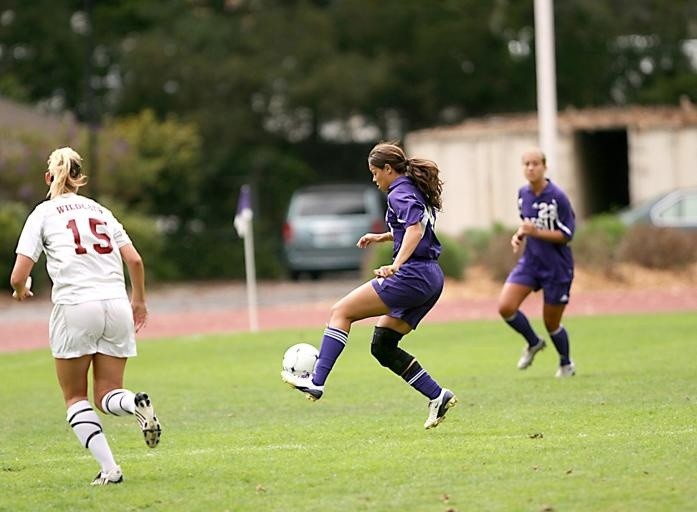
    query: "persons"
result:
[277,138,458,432]
[495,147,579,379]
[8,145,162,487]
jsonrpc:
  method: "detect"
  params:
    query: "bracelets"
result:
[386,231,393,241]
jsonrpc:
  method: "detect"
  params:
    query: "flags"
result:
[233,185,253,240]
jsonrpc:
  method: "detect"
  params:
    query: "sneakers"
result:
[424,386,458,431]
[517,337,546,369]
[89,465,125,488]
[281,370,324,403]
[132,391,162,449]
[554,359,576,378]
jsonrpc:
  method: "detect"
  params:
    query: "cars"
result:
[619,185,695,262]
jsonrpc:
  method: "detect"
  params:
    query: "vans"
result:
[280,183,382,282]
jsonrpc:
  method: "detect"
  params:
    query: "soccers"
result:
[283,343,319,377]
